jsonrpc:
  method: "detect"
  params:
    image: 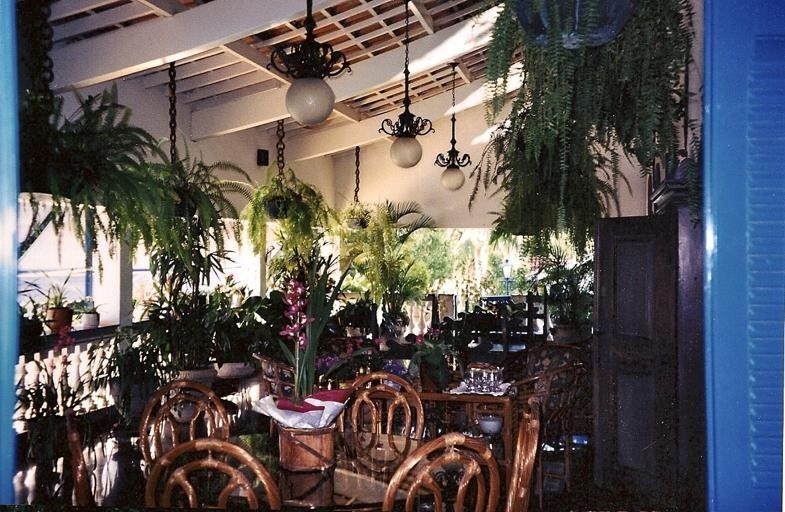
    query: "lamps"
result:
[378,1,474,191]
[270,0,353,129]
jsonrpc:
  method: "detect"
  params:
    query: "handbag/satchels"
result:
[249,386,358,430]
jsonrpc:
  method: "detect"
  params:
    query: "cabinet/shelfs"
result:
[593,159,710,512]
